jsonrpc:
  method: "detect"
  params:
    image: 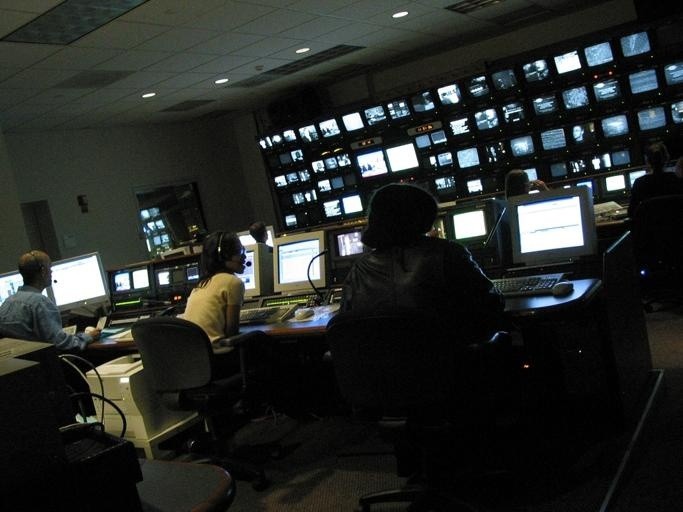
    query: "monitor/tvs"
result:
[584,28,683,199]
[236,224,276,247]
[273,230,326,296]
[508,184,597,266]
[49,251,112,312]
[436,71,500,196]
[130,265,156,299]
[446,199,506,252]
[155,262,200,293]
[234,243,273,300]
[329,225,367,269]
[0,269,49,307]
[139,205,177,252]
[297,112,364,219]
[259,129,297,230]
[500,50,585,194]
[365,90,436,187]
[111,269,130,303]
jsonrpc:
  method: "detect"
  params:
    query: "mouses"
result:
[294,307,315,320]
[552,282,573,297]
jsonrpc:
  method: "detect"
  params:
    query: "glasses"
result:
[232,247,245,256]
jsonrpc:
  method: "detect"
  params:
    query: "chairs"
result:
[624,192,681,309]
[129,317,274,494]
[321,307,510,512]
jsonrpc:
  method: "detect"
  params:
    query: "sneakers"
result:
[248,404,282,420]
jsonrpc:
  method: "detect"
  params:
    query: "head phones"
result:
[29,251,47,276]
[214,233,227,271]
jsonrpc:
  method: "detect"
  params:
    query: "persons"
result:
[0,250,102,422]
[339,183,508,425]
[248,222,272,252]
[183,230,280,417]
[503,169,550,201]
[628,143,683,220]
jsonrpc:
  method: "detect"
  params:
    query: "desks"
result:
[85,263,605,349]
[1,457,237,512]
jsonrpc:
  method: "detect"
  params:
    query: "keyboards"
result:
[239,304,299,324]
[491,273,564,296]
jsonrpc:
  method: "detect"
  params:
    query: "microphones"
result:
[54,280,57,283]
[246,262,251,266]
[54,280,58,283]
